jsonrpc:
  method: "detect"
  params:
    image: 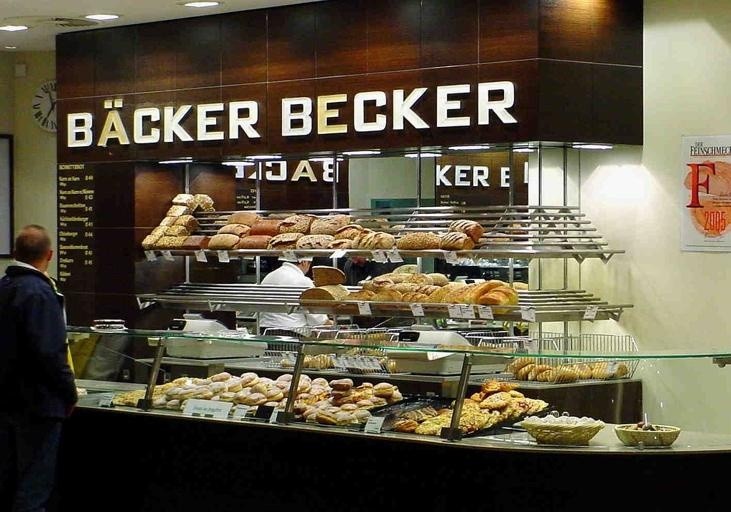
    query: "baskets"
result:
[520,416,607,446]
[615,421,683,448]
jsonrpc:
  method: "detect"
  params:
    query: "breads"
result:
[327,228,396,248]
[296,234,335,249]
[507,357,628,383]
[335,224,363,240]
[293,373,404,425]
[280,354,334,370]
[448,220,484,242]
[392,418,417,432]
[208,233,239,248]
[226,212,259,227]
[310,215,348,235]
[250,221,280,236]
[397,232,439,248]
[684,162,731,238]
[143,194,219,249]
[232,235,272,249]
[115,371,293,416]
[297,264,528,315]
[351,347,396,374]
[438,232,476,249]
[218,223,251,238]
[277,214,317,234]
[414,380,549,434]
[267,233,304,249]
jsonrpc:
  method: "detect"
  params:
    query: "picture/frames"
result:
[0,133,15,259]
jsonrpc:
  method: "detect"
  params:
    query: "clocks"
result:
[31,80,57,133]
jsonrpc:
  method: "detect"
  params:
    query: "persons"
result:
[0,223,79,512]
[346,256,393,329]
[259,255,334,358]
